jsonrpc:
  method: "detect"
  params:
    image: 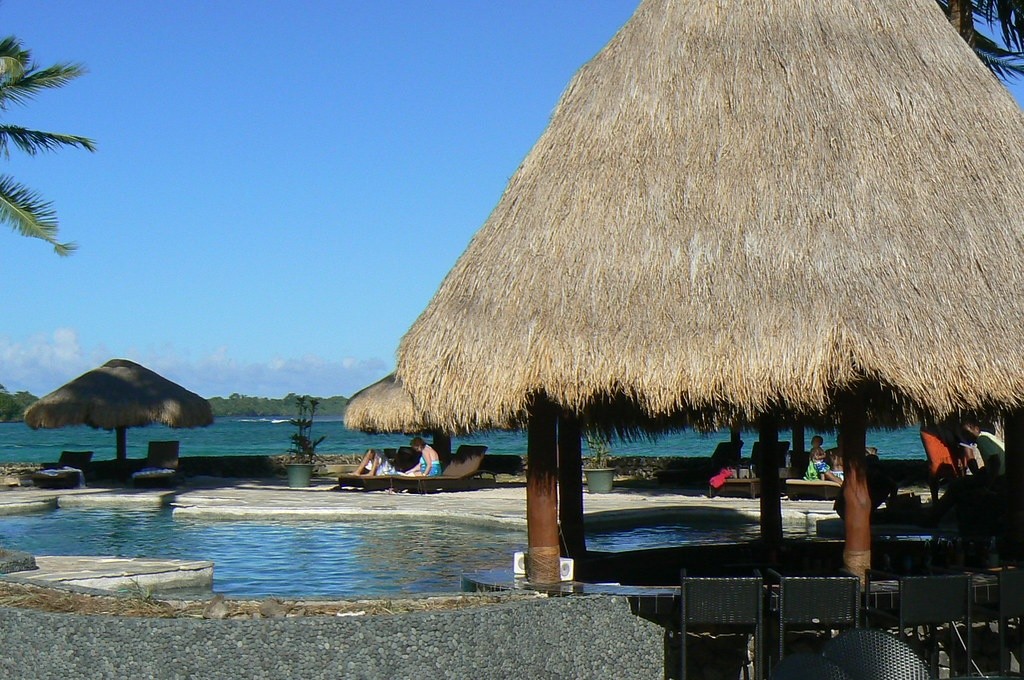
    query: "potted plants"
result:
[285,398,327,489]
[583,430,616,494]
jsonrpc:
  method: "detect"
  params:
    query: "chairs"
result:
[339,445,436,494]
[389,445,497,493]
[132,440,182,488]
[786,447,876,500]
[678,567,1024,680]
[33,452,94,490]
[707,442,790,498]
[654,440,745,497]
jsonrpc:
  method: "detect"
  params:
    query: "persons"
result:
[809,435,830,481]
[824,434,846,486]
[406,437,442,477]
[348,448,397,476]
[959,421,1006,488]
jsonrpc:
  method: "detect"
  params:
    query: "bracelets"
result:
[423,473,428,477]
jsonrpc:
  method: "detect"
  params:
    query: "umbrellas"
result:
[22,359,214,458]
[343,0,1023,583]
